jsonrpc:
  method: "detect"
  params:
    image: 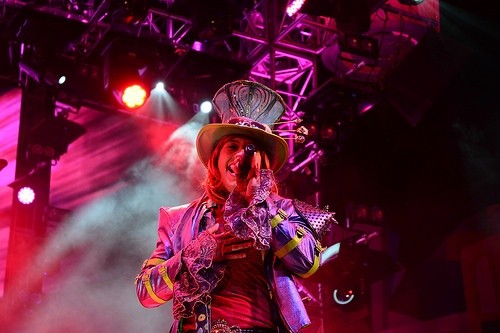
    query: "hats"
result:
[197,80,289,175]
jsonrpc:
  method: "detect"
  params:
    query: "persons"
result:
[135,80,336,333]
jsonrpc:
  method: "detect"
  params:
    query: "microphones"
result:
[238,144,255,176]
